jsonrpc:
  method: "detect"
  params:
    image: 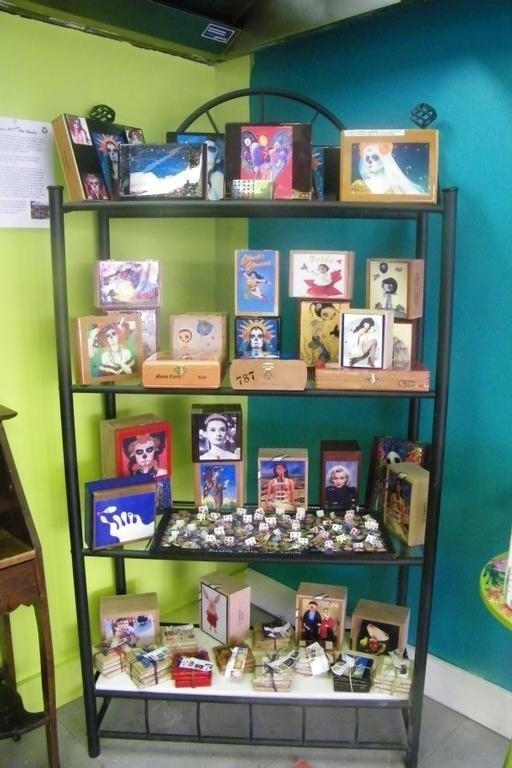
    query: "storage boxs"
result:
[187,396,250,513]
[231,249,284,320]
[101,593,162,647]
[199,569,250,646]
[316,364,429,393]
[294,297,353,372]
[364,256,427,321]
[75,312,145,385]
[140,346,227,391]
[32,180,459,766]
[385,318,425,370]
[221,120,313,202]
[336,306,393,371]
[84,472,158,549]
[167,312,232,363]
[228,358,309,392]
[369,432,431,550]
[229,316,281,358]
[98,415,174,483]
[318,438,361,511]
[257,444,310,518]
[90,256,165,312]
[49,114,226,203]
[309,126,440,205]
[287,250,357,301]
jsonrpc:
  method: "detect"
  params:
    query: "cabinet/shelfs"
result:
[0,408,67,766]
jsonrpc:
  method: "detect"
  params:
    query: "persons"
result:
[348,316,379,367]
[242,264,271,302]
[386,476,409,524]
[197,415,239,460]
[64,116,141,201]
[263,462,297,514]
[325,466,357,509]
[301,601,336,639]
